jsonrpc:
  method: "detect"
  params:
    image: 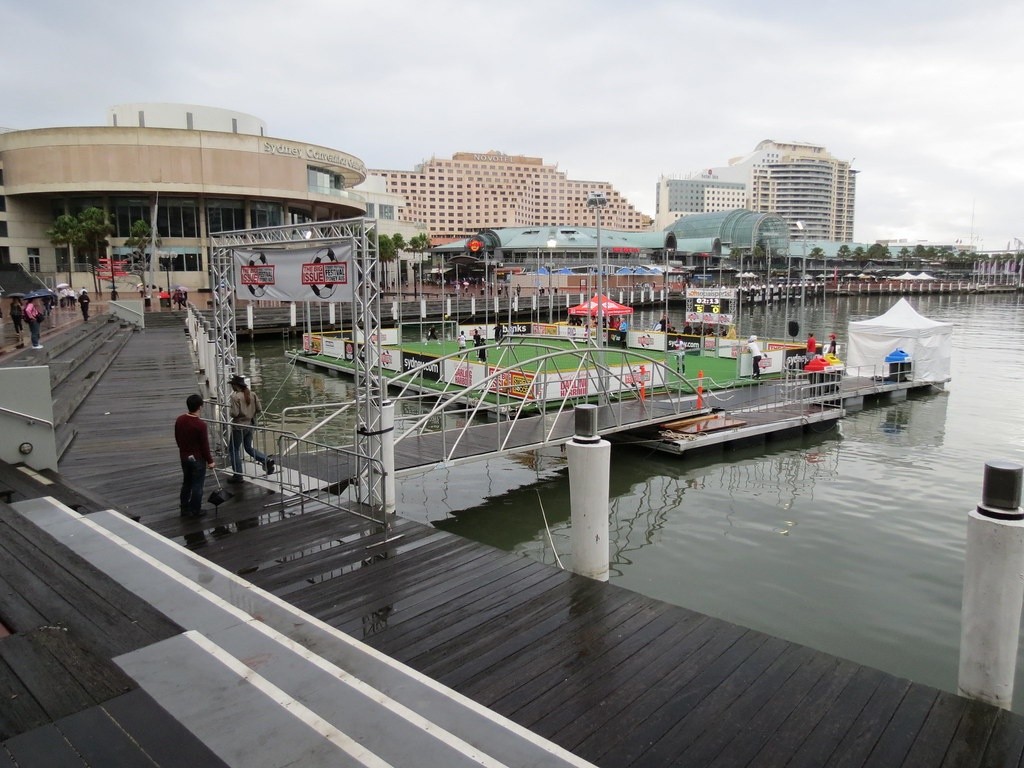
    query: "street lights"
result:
[582,191,608,404]
[546,238,557,325]
[797,220,807,343]
[156,250,178,308]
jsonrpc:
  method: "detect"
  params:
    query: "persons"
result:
[173,289,187,310]
[807,332,836,362]
[425,321,504,363]
[52,287,90,321]
[746,335,762,380]
[140,285,144,297]
[9,297,51,349]
[424,278,558,296]
[228,374,275,482]
[111,288,119,301]
[570,315,627,348]
[659,313,728,376]
[175,394,216,517]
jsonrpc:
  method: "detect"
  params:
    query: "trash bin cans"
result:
[160,298,171,308]
[885,349,912,382]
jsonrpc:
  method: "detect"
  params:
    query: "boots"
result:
[178,496,208,518]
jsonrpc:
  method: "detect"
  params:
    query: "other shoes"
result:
[267,459,274,475]
[478,360,486,364]
[425,343,427,345]
[227,477,243,483]
[438,342,441,344]
[32,344,43,349]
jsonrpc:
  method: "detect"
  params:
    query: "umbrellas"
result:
[137,283,157,289]
[25,289,53,298]
[462,281,469,286]
[57,283,69,289]
[106,285,118,289]
[7,292,25,298]
[177,287,188,292]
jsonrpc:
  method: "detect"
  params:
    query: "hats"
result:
[227,375,246,386]
[830,335,837,339]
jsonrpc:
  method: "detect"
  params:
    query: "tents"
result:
[569,294,632,330]
[848,297,952,379]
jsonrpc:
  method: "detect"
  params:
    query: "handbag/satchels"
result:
[36,312,44,323]
